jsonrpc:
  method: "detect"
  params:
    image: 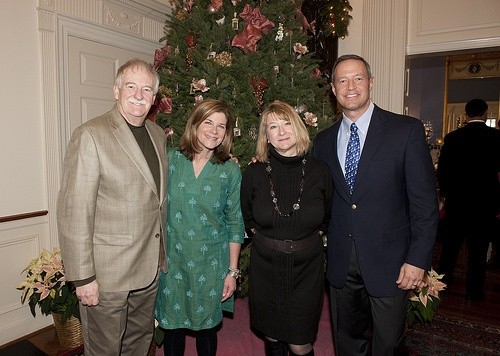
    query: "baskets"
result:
[50,310,84,349]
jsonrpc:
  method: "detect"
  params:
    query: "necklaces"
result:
[266,155,307,216]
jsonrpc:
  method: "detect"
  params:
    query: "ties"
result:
[342,123,361,196]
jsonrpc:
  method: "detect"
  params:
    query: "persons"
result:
[248,55,439,356]
[57,59,241,356]
[241,101,333,356]
[437,99,500,300]
[154,99,243,356]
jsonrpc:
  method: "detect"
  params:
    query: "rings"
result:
[413,284,417,287]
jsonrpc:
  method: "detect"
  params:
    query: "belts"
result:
[255,230,321,253]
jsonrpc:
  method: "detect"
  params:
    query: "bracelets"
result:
[228,268,241,279]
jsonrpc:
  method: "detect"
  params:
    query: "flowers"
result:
[406,267,448,328]
[16,246,82,325]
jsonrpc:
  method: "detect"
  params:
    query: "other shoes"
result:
[465,291,486,303]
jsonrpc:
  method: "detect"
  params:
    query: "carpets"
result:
[397,316,500,356]
